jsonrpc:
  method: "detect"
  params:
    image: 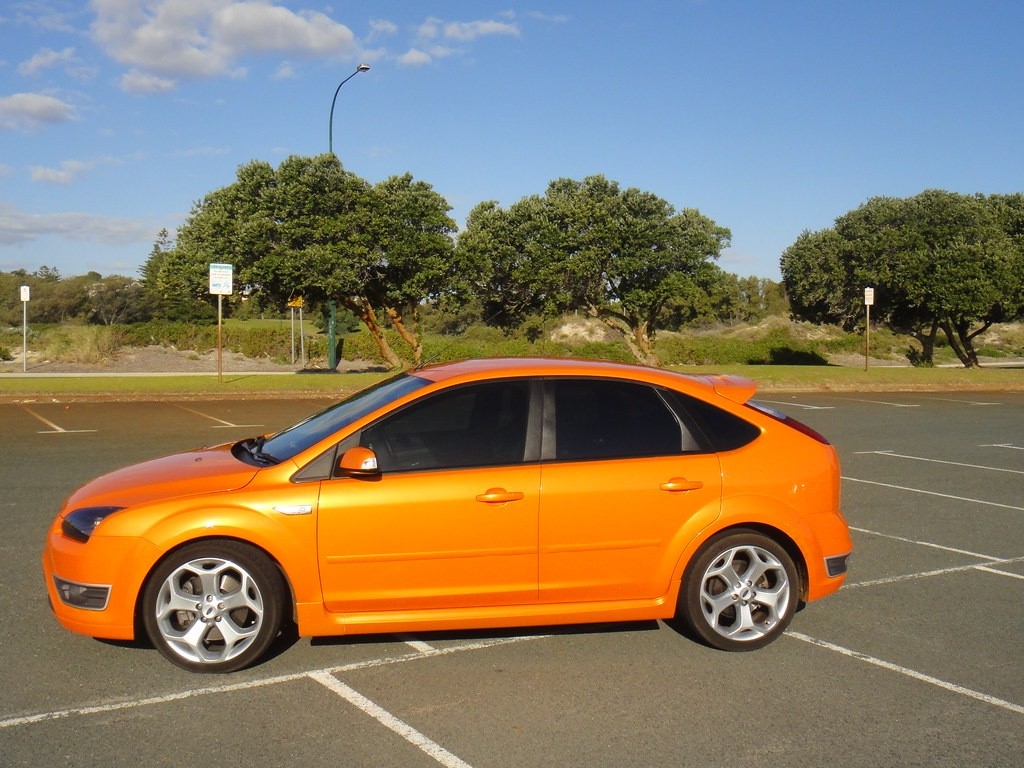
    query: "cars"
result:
[41,357,854,673]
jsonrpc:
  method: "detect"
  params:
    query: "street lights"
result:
[328,64,371,369]
